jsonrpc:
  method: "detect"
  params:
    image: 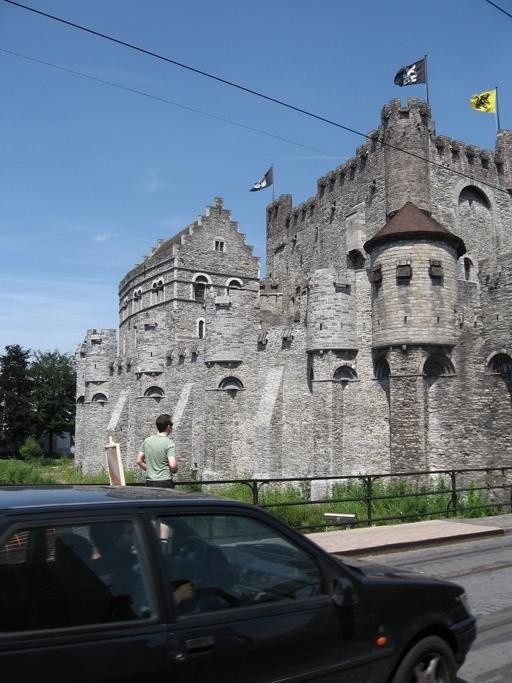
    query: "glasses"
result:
[168,423,173,426]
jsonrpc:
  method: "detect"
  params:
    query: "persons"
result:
[90,521,138,614]
[135,413,179,543]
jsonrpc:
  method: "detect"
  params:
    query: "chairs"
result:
[55,532,93,580]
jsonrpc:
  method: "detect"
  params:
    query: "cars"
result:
[1,485,476,682]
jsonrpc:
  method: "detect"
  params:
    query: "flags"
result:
[394,57,426,87]
[469,89,497,114]
[248,166,273,192]
